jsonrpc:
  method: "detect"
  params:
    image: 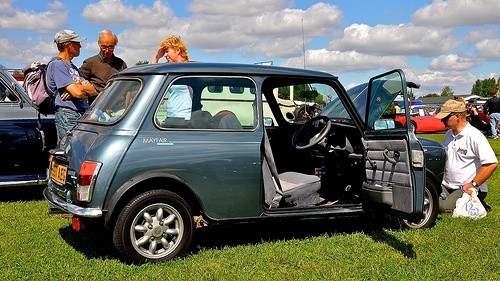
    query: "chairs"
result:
[208,110,322,210]
[191,111,212,129]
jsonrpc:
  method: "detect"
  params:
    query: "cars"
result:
[396,104,448,133]
[0,64,59,189]
[40,60,448,265]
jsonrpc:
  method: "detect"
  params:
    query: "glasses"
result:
[100,46,114,51]
[444,112,457,120]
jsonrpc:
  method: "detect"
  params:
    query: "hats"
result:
[54,30,87,43]
[435,99,468,119]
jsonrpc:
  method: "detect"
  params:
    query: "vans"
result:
[202,81,323,127]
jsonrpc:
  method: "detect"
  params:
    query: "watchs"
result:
[471,180,480,188]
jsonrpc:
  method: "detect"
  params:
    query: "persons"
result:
[482,89,500,139]
[435,99,498,213]
[46,30,96,148]
[152,35,192,118]
[78,30,127,92]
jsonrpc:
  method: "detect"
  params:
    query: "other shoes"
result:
[49,208,64,213]
[493,138,496,140]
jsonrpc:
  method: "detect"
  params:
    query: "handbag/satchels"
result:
[452,188,488,219]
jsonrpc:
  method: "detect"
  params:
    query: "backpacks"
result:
[23,56,70,115]
